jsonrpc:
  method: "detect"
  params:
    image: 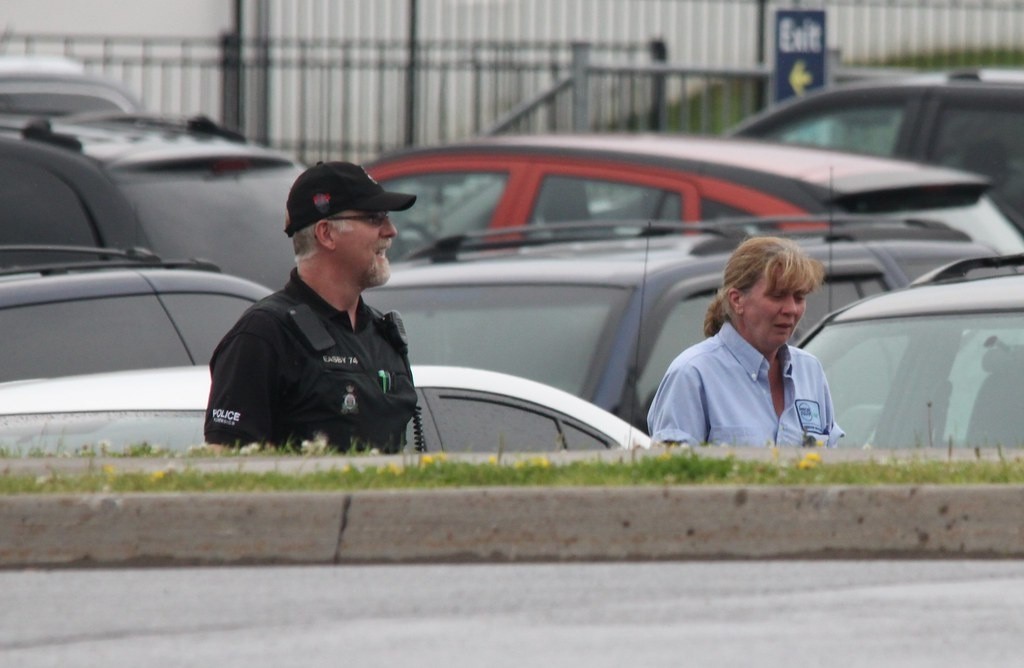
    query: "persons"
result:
[647,235,846,449]
[203,159,417,456]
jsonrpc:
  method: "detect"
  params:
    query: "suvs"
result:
[0,244,278,383]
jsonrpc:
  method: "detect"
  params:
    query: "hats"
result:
[283,159,417,238]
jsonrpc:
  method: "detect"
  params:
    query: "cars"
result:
[368,212,1023,438]
[587,65,1024,248]
[2,366,655,461]
[2,63,316,293]
[333,134,1023,286]
[790,278,1024,457]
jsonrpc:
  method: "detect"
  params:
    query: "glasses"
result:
[328,212,386,224]
